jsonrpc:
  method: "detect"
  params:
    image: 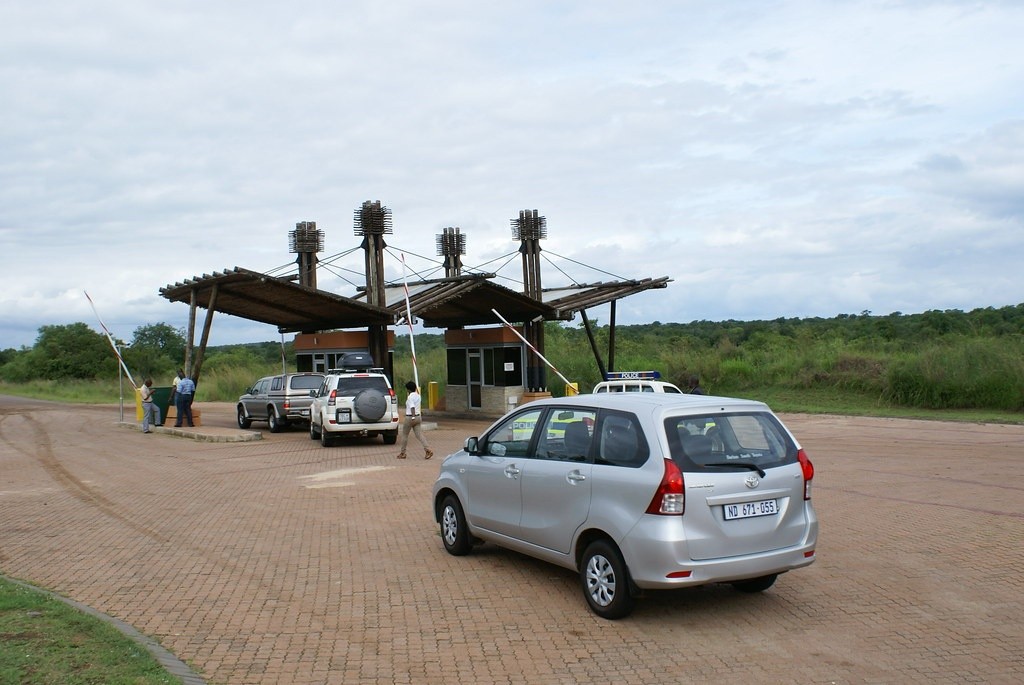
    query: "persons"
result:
[140,379,163,433]
[396,381,433,459]
[168,368,196,427]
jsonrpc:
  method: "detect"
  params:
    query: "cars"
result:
[430,391,821,620]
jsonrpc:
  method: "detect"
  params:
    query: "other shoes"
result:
[189,424,194,427]
[175,424,182,427]
[155,424,164,427]
[145,430,153,434]
[424,450,433,458]
[396,453,407,459]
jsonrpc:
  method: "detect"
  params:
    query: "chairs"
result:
[565,422,589,459]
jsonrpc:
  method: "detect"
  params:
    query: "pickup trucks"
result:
[509,369,715,441]
[235,370,326,433]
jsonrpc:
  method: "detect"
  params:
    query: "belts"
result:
[406,414,419,417]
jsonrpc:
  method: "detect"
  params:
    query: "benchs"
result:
[681,434,712,457]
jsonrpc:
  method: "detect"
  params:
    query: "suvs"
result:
[308,352,400,447]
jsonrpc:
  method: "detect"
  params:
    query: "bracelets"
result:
[412,417,415,419]
